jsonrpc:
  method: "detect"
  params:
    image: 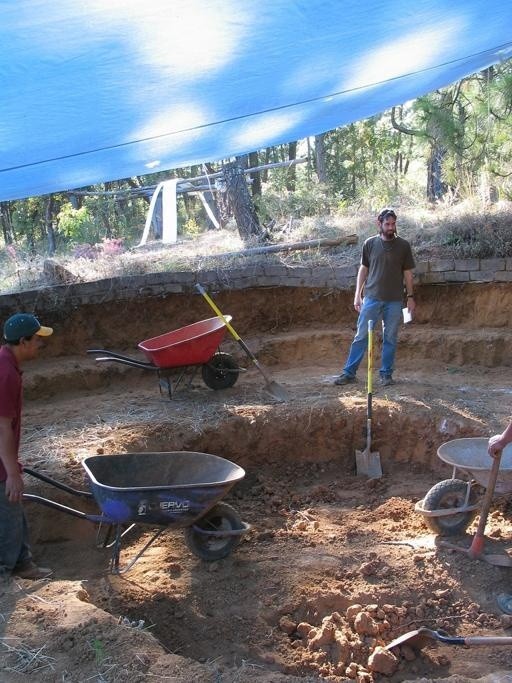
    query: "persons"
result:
[487,421,512,615]
[333,210,416,387]
[0,312,54,582]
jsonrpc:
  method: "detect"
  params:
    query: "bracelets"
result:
[407,294,414,297]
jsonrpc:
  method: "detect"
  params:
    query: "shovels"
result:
[385,627,512,652]
[195,283,290,402]
[354,320,383,479]
[438,541,510,571]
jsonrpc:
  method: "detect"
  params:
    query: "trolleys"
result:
[17,448,251,575]
[86,312,241,400]
[414,428,512,538]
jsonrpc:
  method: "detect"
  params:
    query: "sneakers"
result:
[14,566,54,579]
[334,374,356,385]
[380,375,393,386]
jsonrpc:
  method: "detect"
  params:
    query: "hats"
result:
[3,313,54,342]
